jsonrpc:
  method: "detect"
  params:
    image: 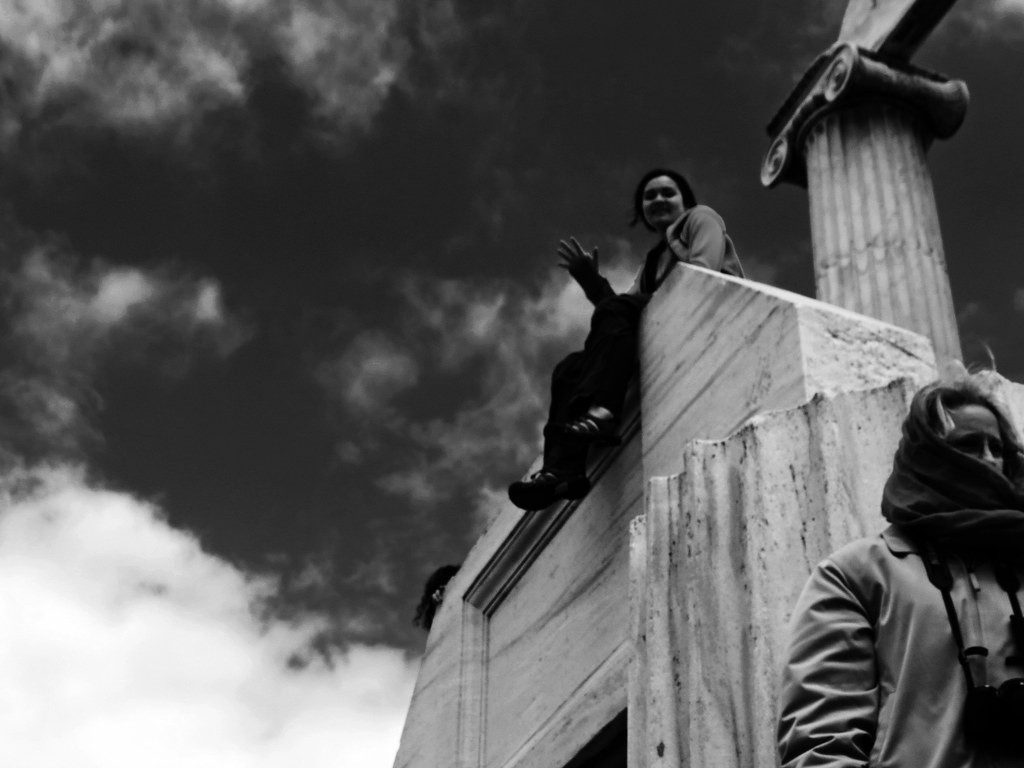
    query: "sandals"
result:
[557,413,622,447]
[508,464,593,511]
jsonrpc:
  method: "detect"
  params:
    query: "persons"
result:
[776,379,1024,768]
[507,166,743,511]
